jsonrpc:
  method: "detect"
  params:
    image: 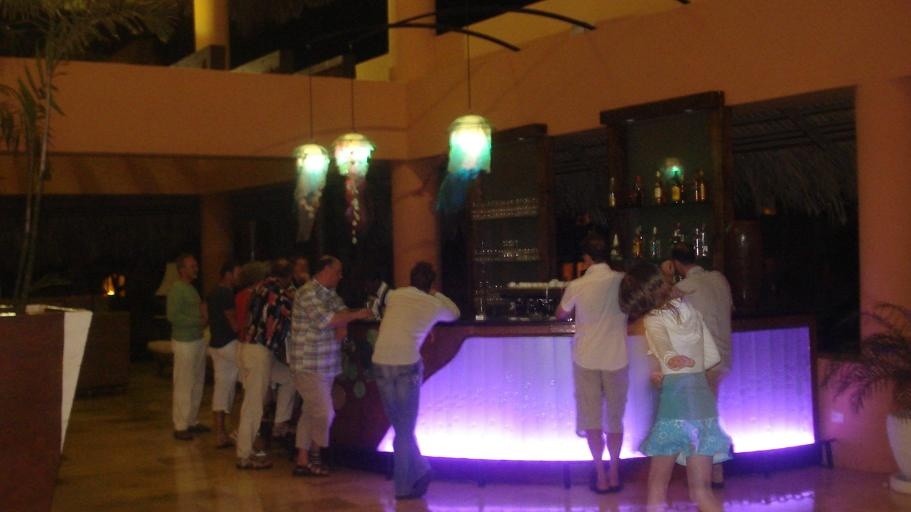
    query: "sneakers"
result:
[711,481,725,487]
[395,495,417,500]
[174,431,193,441]
[293,464,332,477]
[415,470,432,497]
[236,455,272,470]
[189,424,208,433]
[271,424,297,440]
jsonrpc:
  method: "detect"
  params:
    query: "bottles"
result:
[694,167,706,201]
[693,226,703,261]
[632,226,648,261]
[608,177,618,208]
[632,175,645,205]
[701,221,710,258]
[671,170,685,204]
[654,171,666,205]
[649,226,663,259]
[671,222,685,248]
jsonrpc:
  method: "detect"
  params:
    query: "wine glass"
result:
[476,238,540,259]
[470,196,540,219]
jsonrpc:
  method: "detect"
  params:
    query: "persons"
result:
[225,258,297,470]
[671,241,736,490]
[166,253,212,441]
[618,261,736,511]
[354,267,394,322]
[208,262,274,449]
[284,255,375,478]
[232,261,272,457]
[553,237,632,494]
[289,256,311,291]
[366,260,463,500]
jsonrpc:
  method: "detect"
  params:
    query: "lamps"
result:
[333,1,377,244]
[431,1,491,216]
[155,260,183,295]
[291,0,333,240]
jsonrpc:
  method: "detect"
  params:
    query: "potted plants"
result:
[821,302,911,494]
[0,1,191,512]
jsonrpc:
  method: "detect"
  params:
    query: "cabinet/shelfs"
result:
[599,89,726,285]
[471,123,554,321]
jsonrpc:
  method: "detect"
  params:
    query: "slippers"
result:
[609,480,622,492]
[590,477,609,494]
